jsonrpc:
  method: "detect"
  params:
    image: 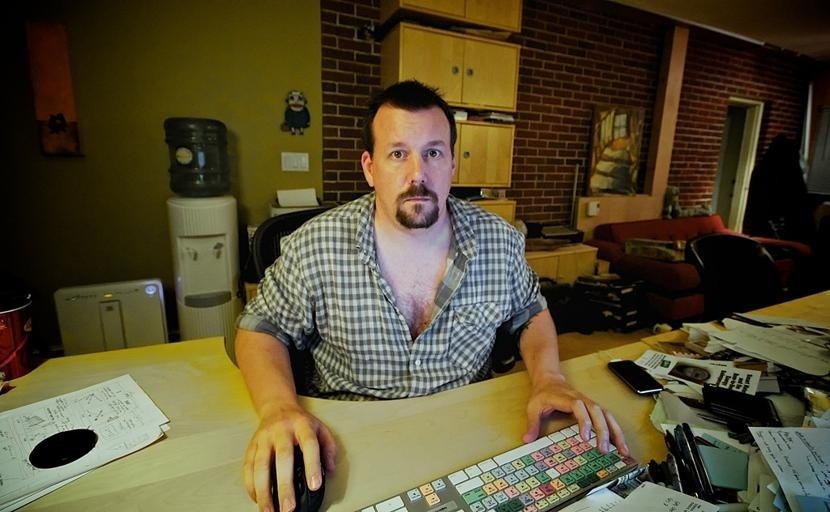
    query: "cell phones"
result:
[607,360,664,395]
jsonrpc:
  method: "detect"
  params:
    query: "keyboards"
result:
[357,415,641,511]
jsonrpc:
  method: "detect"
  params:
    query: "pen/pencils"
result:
[649,422,716,505]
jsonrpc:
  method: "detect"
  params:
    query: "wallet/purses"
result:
[702,382,783,426]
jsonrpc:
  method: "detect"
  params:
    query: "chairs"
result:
[686,232,779,320]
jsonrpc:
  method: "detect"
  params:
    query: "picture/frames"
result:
[581,102,654,197]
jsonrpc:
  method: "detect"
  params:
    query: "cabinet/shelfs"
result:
[450,119,516,189]
[468,200,517,227]
[375,0,524,39]
[524,249,558,283]
[379,21,523,120]
[553,243,598,284]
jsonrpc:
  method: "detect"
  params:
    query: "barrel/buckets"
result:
[162,117,230,199]
[0,292,34,381]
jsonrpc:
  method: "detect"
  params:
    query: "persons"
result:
[234,80,630,512]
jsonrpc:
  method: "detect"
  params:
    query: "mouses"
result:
[270,442,327,511]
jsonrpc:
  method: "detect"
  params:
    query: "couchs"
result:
[585,213,811,320]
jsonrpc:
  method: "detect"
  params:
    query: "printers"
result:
[268,198,338,218]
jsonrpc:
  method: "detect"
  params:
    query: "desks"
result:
[0,287,829,512]
